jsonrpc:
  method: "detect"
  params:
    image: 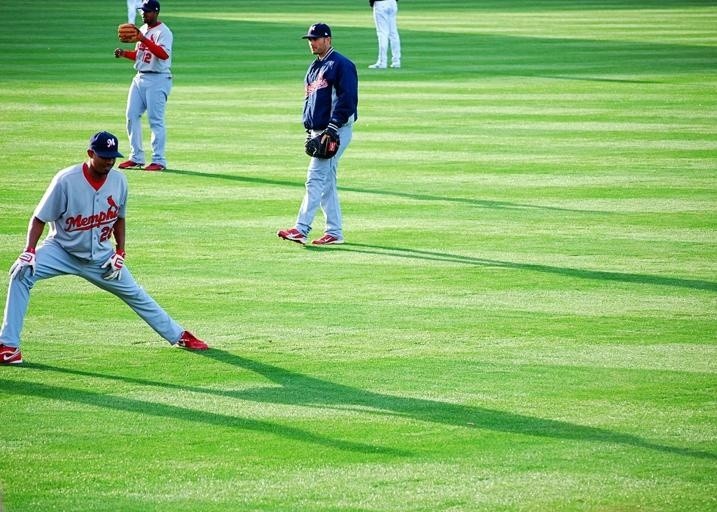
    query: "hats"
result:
[135,1,160,12]
[89,131,123,159]
[301,24,331,40]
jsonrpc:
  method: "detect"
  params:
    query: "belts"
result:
[140,71,160,74]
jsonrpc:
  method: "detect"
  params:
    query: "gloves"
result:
[304,140,314,157]
[113,48,123,59]
[8,247,36,282]
[98,249,125,282]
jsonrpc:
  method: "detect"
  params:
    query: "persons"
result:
[114,0,174,171]
[277,23,358,246]
[0,131,209,365]
[125,0,151,26]
[369,0,402,68]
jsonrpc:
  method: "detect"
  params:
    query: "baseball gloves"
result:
[118,25,143,44]
[305,128,339,158]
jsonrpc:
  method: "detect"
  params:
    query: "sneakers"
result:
[175,330,208,351]
[276,228,306,245]
[144,163,166,171]
[368,64,387,70]
[0,343,22,365]
[389,64,400,68]
[311,234,344,245]
[118,160,145,170]
